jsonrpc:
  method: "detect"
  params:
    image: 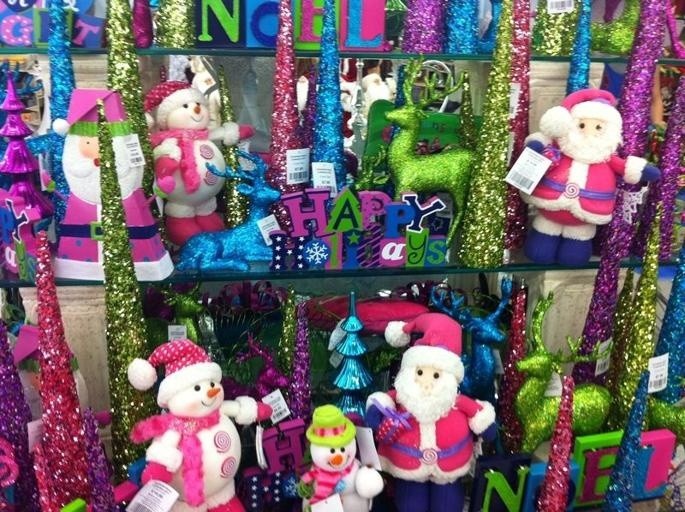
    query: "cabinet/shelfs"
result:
[0,1,683,511]
